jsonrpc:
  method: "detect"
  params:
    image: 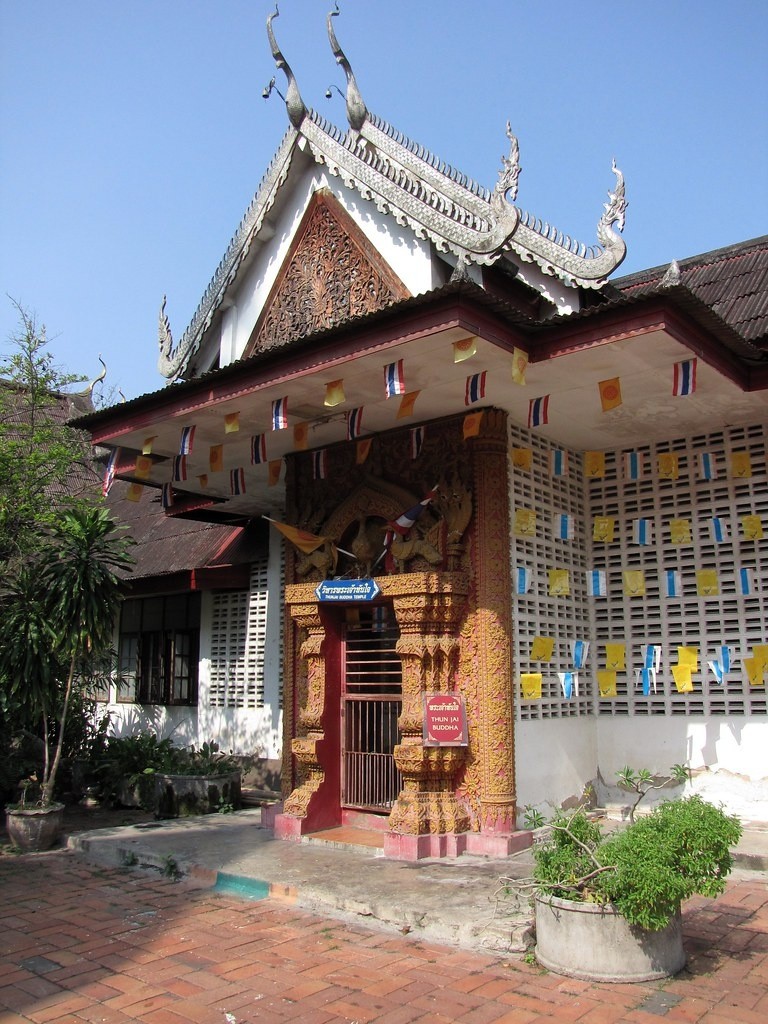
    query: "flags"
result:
[126,435,156,503]
[199,396,288,495]
[514,448,768,699]
[673,358,696,397]
[528,394,550,429]
[512,348,528,386]
[160,424,196,507]
[453,335,487,440]
[102,448,120,498]
[599,377,623,413]
[293,358,425,479]
[274,521,339,573]
[382,491,438,571]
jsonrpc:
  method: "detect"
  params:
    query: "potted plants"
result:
[64,714,254,821]
[484,764,740,982]
[0,505,139,854]
